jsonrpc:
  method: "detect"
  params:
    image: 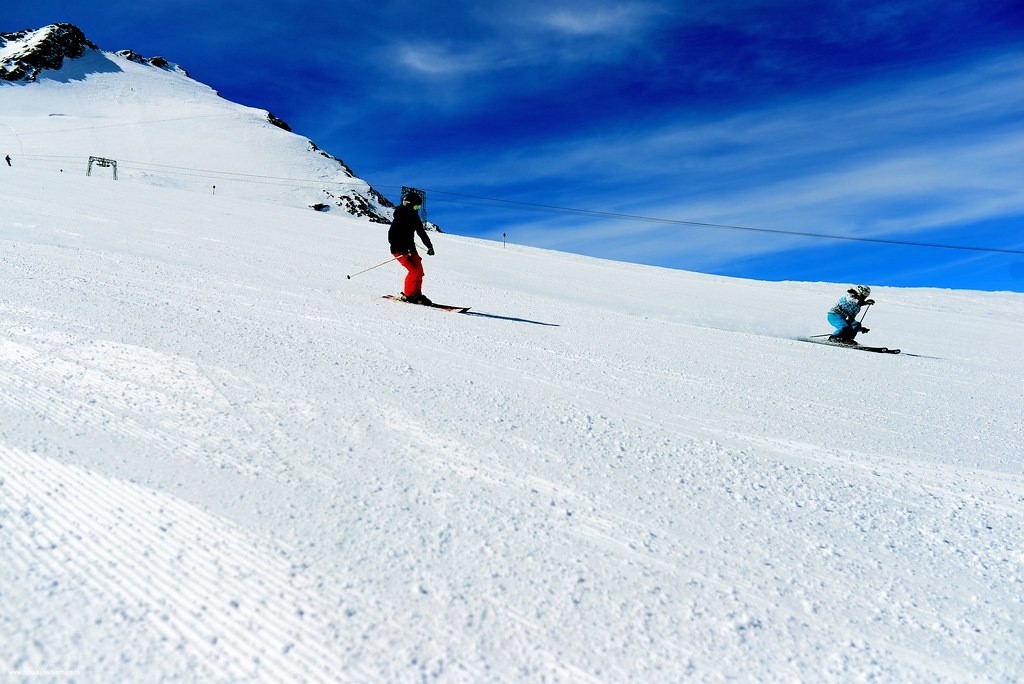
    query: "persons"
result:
[5,155,12,166]
[827,284,875,345]
[387,192,435,304]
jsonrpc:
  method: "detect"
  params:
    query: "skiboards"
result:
[797,337,901,354]
[383,293,472,313]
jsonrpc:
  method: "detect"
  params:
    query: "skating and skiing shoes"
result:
[402,293,428,305]
[830,336,857,346]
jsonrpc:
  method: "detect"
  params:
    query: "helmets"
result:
[402,192,422,212]
[854,284,871,298]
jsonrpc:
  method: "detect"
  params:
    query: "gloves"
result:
[426,248,436,256]
[860,327,869,333]
[865,299,875,306]
